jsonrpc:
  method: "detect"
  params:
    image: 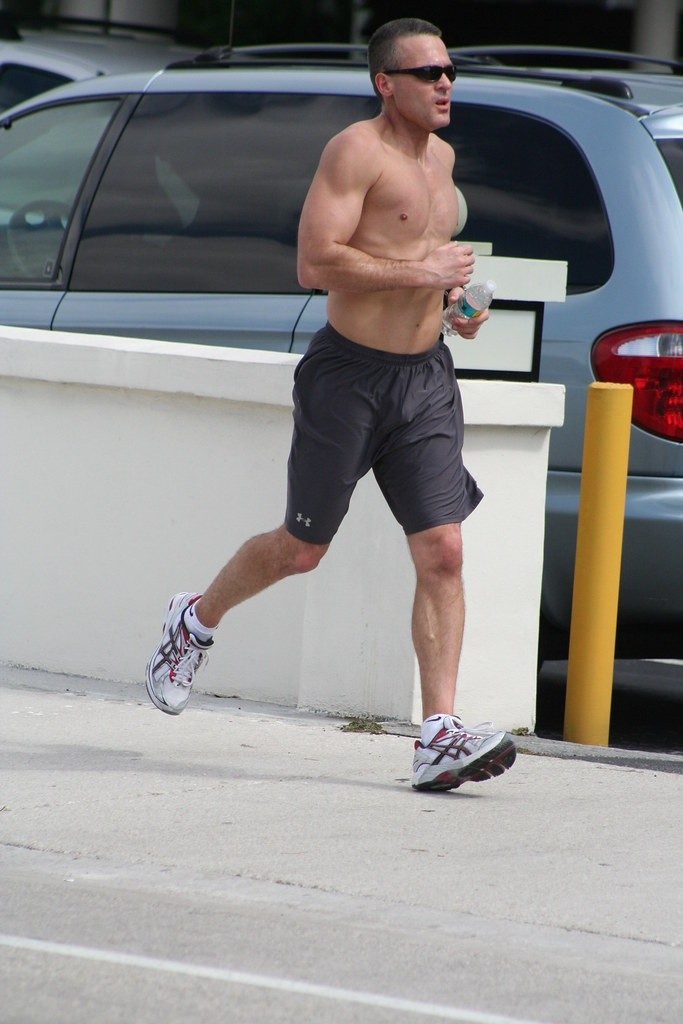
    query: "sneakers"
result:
[146,593,216,715]
[410,715,517,792]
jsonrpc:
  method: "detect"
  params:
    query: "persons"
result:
[140,18,518,792]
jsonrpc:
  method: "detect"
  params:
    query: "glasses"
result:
[383,63,458,83]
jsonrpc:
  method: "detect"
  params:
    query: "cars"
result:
[0,31,203,230]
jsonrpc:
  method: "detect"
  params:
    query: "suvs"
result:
[1,34,683,687]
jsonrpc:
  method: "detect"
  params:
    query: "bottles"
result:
[440,280,496,337]
